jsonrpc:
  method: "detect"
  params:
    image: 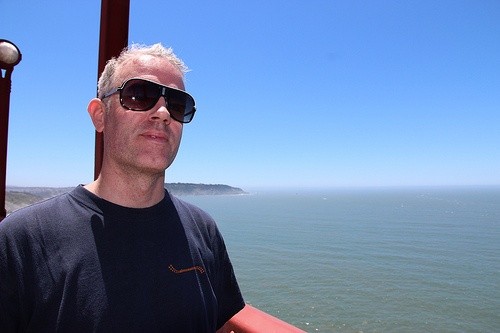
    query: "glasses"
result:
[101,78,195,124]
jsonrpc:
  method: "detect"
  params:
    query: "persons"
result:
[0,42,308,333]
